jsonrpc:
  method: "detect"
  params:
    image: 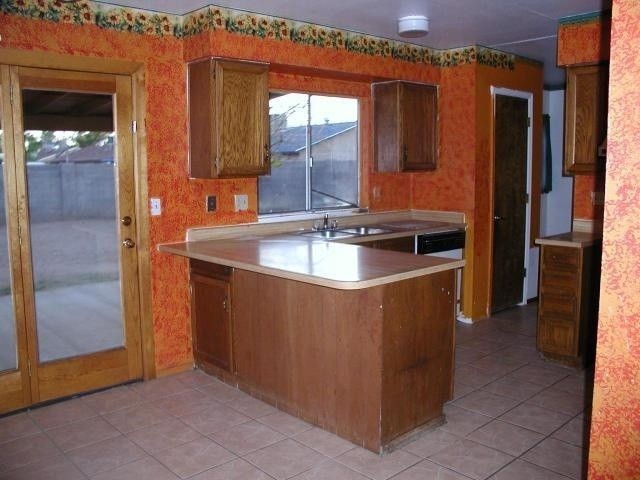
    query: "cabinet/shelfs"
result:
[187,60,272,179]
[562,66,607,179]
[190,261,234,385]
[366,237,416,260]
[370,81,438,174]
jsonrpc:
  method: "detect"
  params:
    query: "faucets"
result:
[323,214,329,228]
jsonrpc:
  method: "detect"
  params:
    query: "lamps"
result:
[398,16,429,38]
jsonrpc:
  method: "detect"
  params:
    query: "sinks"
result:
[289,229,363,241]
[340,226,396,236]
